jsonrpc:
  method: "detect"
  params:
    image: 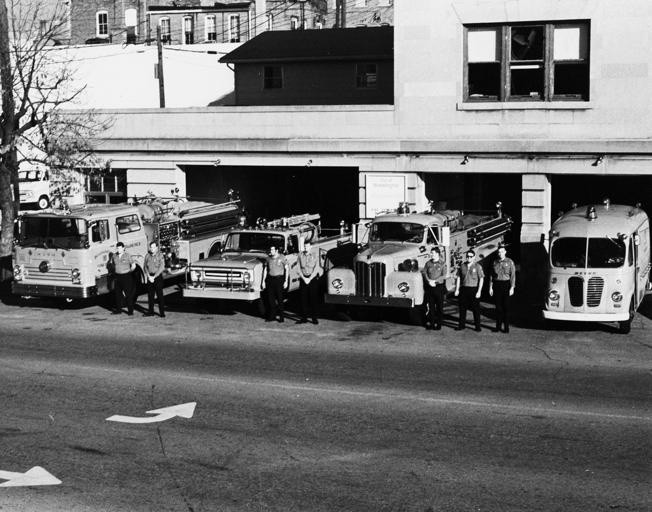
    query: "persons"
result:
[488,246,515,333]
[261,243,289,322]
[295,240,319,324]
[454,250,485,331]
[422,247,448,330]
[110,242,136,315]
[142,242,165,317]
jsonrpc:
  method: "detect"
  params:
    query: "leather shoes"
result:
[113,308,165,317]
[266,317,318,324]
[425,324,509,333]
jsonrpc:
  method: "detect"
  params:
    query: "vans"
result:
[542,198,652,334]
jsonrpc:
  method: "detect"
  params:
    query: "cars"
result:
[18,170,55,210]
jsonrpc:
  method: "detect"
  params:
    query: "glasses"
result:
[467,255,473,258]
